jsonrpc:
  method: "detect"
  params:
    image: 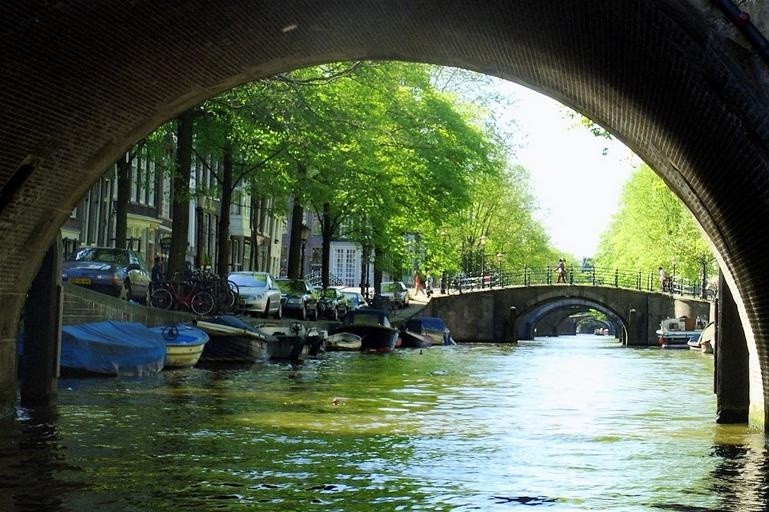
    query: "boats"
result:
[16,319,166,380]
[592,328,610,336]
[655,315,715,355]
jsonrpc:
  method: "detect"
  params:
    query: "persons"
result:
[554,259,566,283]
[658,267,667,292]
[414,270,434,298]
[151,256,163,307]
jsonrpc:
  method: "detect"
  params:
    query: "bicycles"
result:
[149,266,242,317]
[365,292,404,317]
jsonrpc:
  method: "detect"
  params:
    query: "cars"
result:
[226,270,369,321]
[381,282,410,308]
[59,244,153,306]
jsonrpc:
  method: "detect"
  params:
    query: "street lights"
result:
[479,234,487,289]
[192,185,207,269]
[439,227,450,296]
[672,255,678,280]
[496,252,504,285]
[301,220,313,280]
[366,243,374,302]
